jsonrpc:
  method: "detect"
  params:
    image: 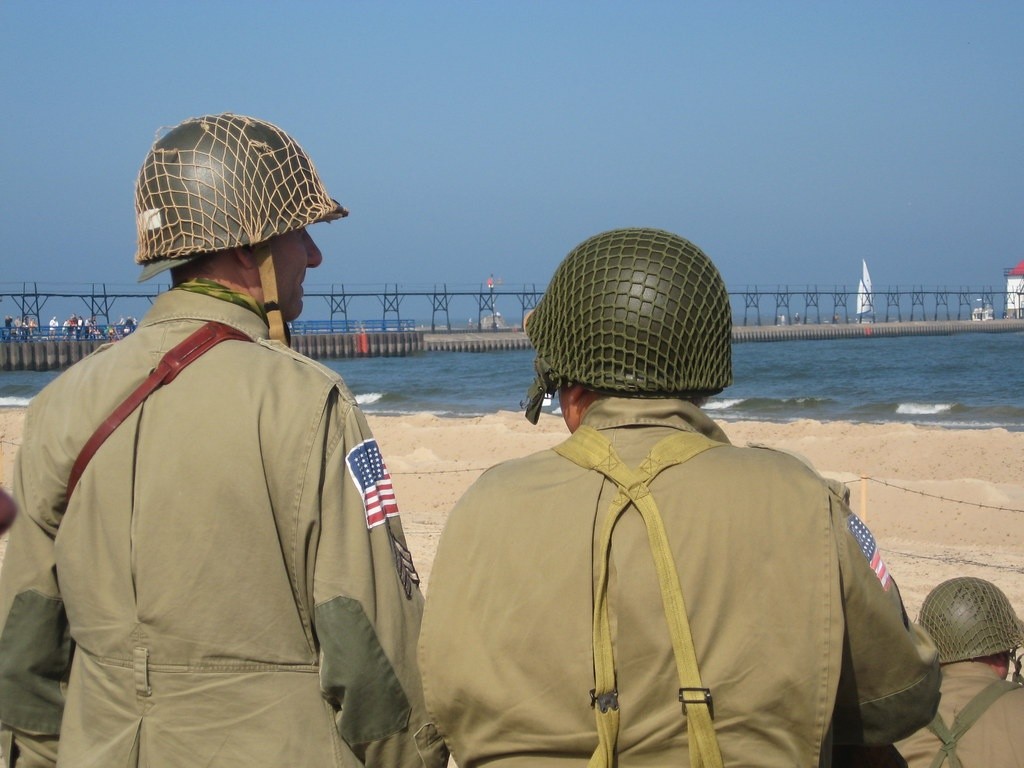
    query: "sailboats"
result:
[856,259,874,323]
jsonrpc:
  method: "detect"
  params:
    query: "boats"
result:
[971,304,994,321]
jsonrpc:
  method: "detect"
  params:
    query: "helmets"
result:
[134,113,349,265]
[524,226,733,392]
[921,577,1024,663]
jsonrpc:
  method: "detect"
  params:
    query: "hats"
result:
[53,316,57,320]
[140,256,196,281]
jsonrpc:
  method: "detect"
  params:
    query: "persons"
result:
[794,313,800,325]
[847,576,1024,768]
[417,227,942,768]
[834,312,840,324]
[0,113,450,768]
[778,314,786,327]
[4,314,137,340]
